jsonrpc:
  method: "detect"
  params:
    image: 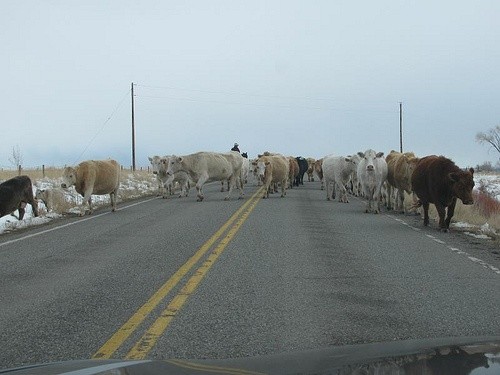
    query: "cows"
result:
[250,152,306,199]
[61,159,120,217]
[148,151,250,201]
[306,149,475,234]
[0,175,40,221]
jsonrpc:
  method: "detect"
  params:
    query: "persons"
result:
[231,143,240,153]
